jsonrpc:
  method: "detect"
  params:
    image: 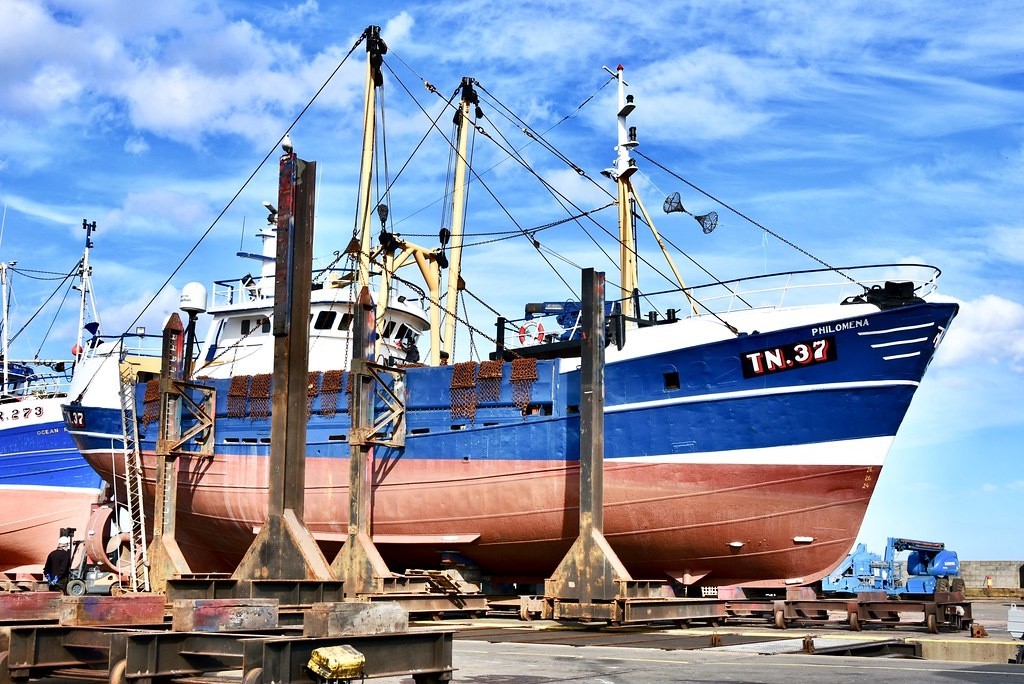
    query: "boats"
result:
[1,196,104,592]
[62,25,961,598]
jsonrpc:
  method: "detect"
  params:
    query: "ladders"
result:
[120,372,151,593]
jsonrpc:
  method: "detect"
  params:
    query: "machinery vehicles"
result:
[821,536,967,601]
[55,482,128,596]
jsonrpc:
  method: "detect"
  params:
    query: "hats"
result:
[59,536,69,545]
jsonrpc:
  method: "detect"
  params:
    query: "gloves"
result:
[45,574,50,582]
[51,575,58,586]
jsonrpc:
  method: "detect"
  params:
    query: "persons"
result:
[44,536,71,595]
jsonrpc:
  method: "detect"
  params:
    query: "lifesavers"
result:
[518,320,544,347]
